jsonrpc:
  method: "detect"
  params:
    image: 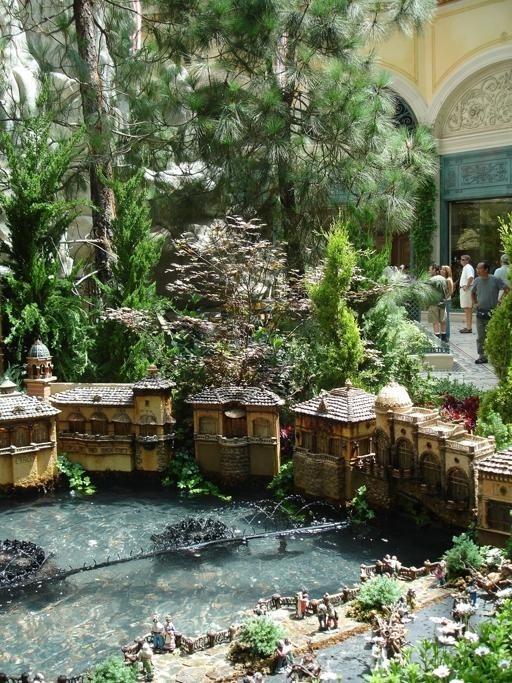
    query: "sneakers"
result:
[443,337,449,342]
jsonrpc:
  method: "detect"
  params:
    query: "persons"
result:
[295,589,338,632]
[343,586,349,603]
[389,263,454,344]
[466,580,479,609]
[460,255,475,333]
[434,560,447,586]
[151,616,175,652]
[407,588,416,609]
[125,638,155,682]
[383,554,399,575]
[275,638,298,668]
[494,254,510,300]
[470,261,510,364]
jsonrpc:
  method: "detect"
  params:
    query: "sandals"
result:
[475,358,488,364]
[460,328,472,333]
